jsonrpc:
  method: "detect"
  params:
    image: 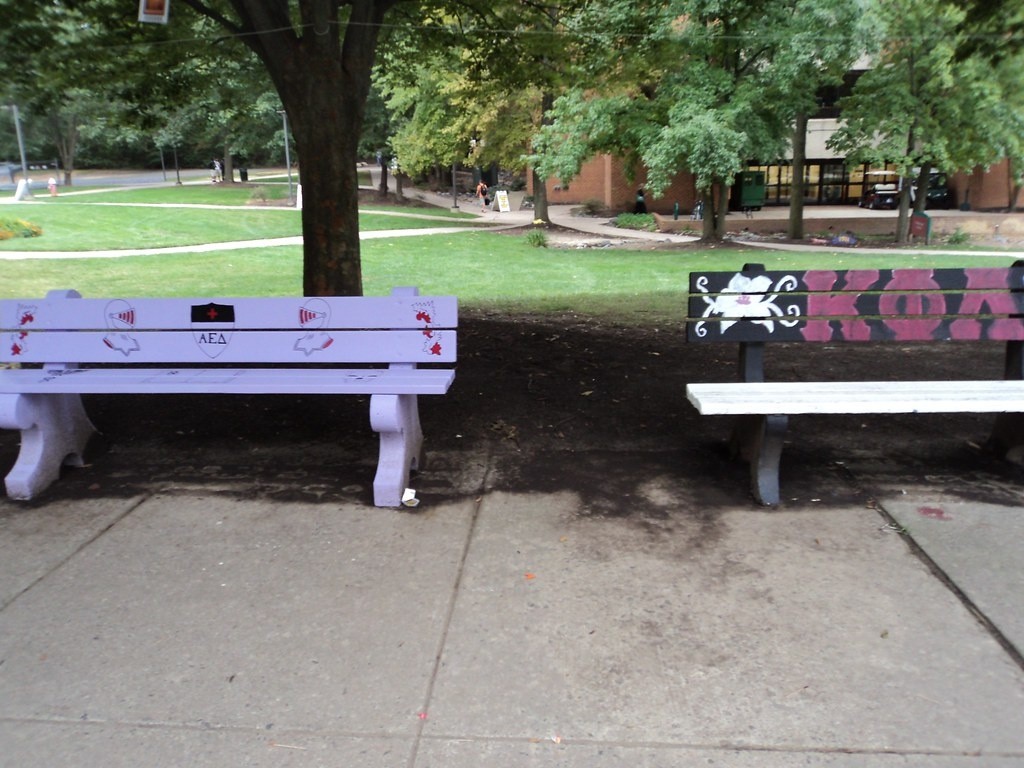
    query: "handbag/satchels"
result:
[637,195,644,202]
[485,198,489,205]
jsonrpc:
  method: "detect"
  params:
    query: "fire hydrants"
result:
[47,178,56,196]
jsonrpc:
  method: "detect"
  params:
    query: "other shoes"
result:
[482,209,486,213]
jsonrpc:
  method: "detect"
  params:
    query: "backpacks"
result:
[479,184,488,196]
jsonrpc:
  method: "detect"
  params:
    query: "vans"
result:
[899,166,954,211]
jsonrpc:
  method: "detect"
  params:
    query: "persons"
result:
[220,157,224,180]
[376,152,382,167]
[209,158,217,182]
[475,179,488,213]
[214,158,221,181]
[634,183,649,215]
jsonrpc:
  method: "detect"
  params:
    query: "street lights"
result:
[276,110,295,207]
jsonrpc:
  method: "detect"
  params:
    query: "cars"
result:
[857,184,901,210]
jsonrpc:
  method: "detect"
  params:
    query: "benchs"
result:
[0,286,459,508]
[682,259,1024,511]
